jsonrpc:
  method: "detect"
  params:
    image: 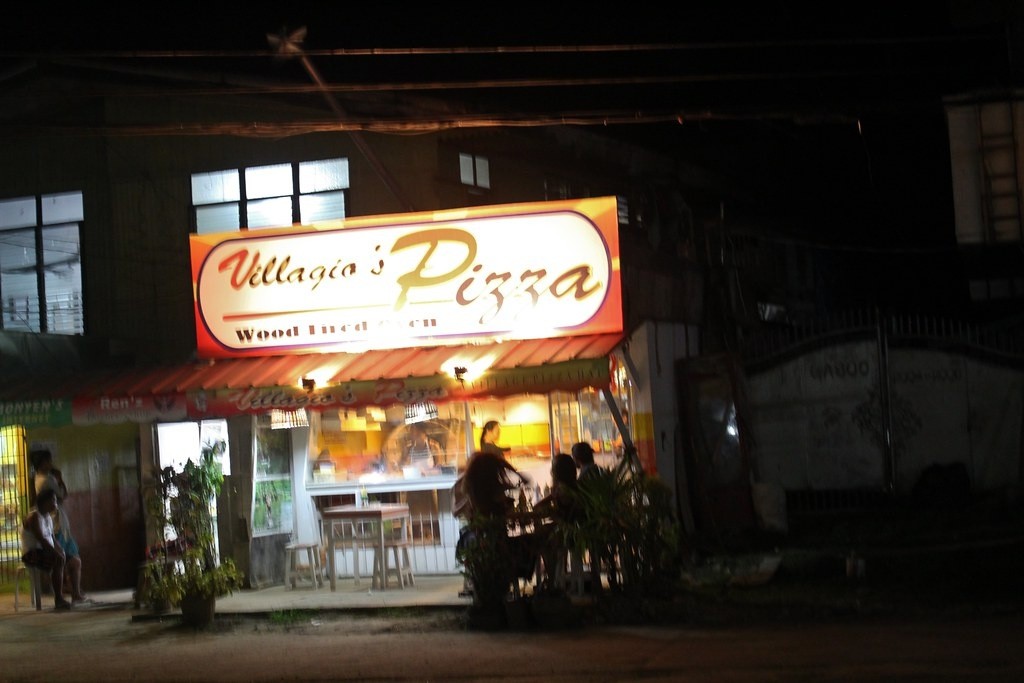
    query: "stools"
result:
[285,543,322,593]
[13,566,50,613]
[370,539,414,591]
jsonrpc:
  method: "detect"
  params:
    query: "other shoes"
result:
[72,595,93,608]
[55,599,71,609]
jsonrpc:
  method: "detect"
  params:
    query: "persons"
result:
[550,453,577,493]
[572,442,606,477]
[391,423,446,472]
[480,421,507,461]
[21,449,87,610]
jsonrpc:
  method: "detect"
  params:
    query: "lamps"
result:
[454,366,468,383]
[302,378,316,394]
[271,407,310,430]
[405,402,438,426]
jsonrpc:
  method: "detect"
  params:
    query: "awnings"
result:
[0,333,643,428]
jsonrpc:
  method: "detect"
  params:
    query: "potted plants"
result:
[133,465,180,614]
[551,463,680,626]
[523,510,575,629]
[166,439,245,628]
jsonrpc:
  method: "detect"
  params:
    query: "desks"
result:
[507,511,550,593]
[322,503,410,592]
